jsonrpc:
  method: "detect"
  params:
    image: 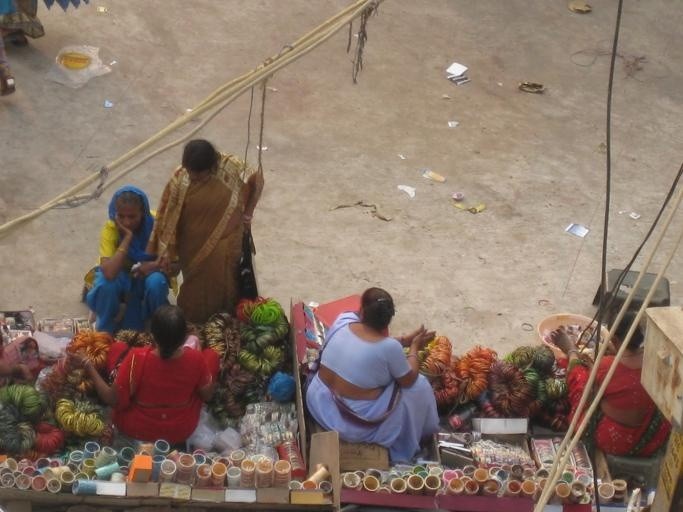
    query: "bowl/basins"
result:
[537,313,611,359]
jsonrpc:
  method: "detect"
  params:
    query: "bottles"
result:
[0,439,628,506]
[442,404,479,433]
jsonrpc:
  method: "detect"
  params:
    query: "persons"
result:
[302,287,439,467]
[66,303,219,445]
[550,310,670,458]
[80,184,180,335]
[145,139,265,324]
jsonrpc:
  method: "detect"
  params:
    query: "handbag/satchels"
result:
[238,258,258,300]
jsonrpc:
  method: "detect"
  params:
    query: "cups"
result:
[183,335,199,350]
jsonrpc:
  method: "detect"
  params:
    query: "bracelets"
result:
[80,357,91,368]
[568,349,579,354]
[399,336,403,345]
[243,214,252,224]
[407,352,418,360]
[117,246,128,256]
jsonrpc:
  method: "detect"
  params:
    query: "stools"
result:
[592,269,670,323]
[606,450,662,489]
[313,294,389,337]
